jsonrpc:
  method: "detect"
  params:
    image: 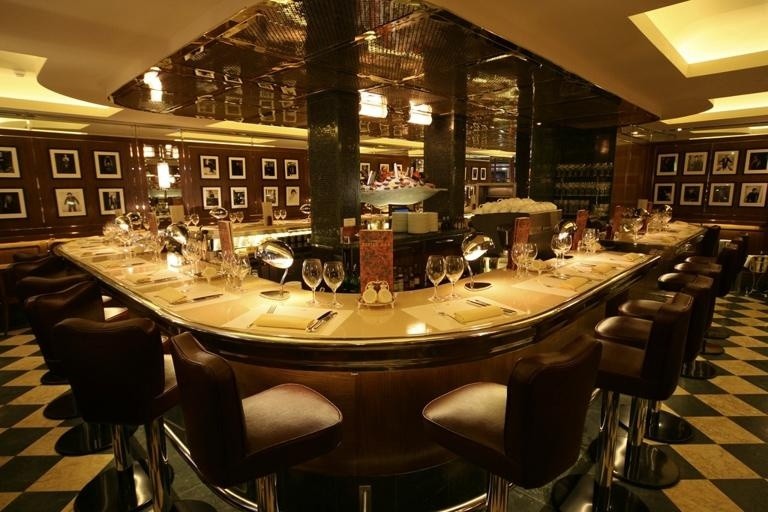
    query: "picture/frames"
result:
[97,187,127,217]
[53,187,87,218]
[0,187,28,220]
[360,162,370,180]
[0,145,22,179]
[199,155,300,210]
[396,164,402,172]
[463,167,487,200]
[48,147,83,180]
[93,150,123,180]
[379,164,389,175]
[653,147,768,208]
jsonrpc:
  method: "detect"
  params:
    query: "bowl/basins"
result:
[357,186,448,206]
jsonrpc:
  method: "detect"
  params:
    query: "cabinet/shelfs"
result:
[554,175,614,218]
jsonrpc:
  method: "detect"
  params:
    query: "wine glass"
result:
[280,209,287,224]
[322,261,345,309]
[101,210,251,292]
[273,210,281,224]
[424,254,447,303]
[444,255,465,300]
[301,258,323,307]
[511,208,672,280]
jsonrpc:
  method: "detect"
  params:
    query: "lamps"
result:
[404,111,433,126]
[157,160,171,189]
[358,104,389,119]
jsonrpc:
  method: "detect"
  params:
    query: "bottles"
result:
[363,283,378,304]
[377,282,393,304]
[343,264,423,291]
[553,162,615,217]
[441,212,465,232]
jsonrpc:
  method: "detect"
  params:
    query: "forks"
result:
[246,305,277,327]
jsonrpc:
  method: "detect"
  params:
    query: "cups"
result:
[360,213,390,230]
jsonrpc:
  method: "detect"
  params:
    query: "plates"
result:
[390,211,439,235]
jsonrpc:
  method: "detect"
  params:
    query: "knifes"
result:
[306,310,338,332]
[171,293,224,305]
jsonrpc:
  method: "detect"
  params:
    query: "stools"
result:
[14,226,128,423]
[594,276,716,486]
[616,234,750,443]
[159,330,343,511]
[551,291,693,512]
[419,340,601,511]
[54,312,174,512]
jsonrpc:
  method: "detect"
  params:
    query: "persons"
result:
[61,154,69,168]
[64,193,80,212]
[746,187,758,203]
[109,193,116,209]
[719,155,733,169]
[288,189,298,202]
